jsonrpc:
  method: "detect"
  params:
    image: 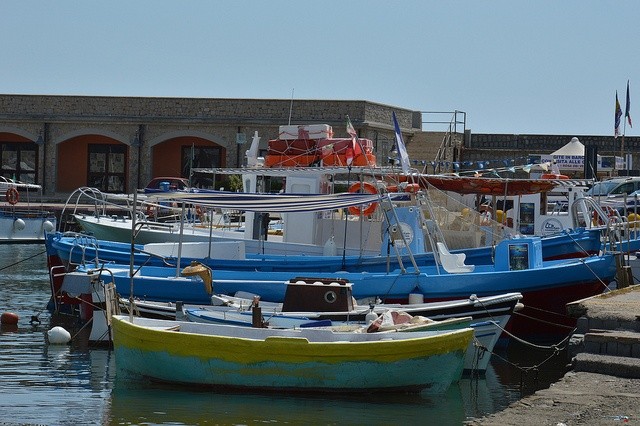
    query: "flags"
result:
[393,111,411,177]
[345,113,358,152]
[615,90,623,138]
[625,80,633,128]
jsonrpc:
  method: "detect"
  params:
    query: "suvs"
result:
[585,177,640,207]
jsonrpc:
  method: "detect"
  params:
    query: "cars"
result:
[144,176,199,193]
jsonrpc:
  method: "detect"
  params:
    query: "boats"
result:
[112,315,473,387]
[53,233,601,309]
[383,178,640,253]
[212,292,523,311]
[72,164,517,292]
[185,308,474,327]
[51,209,618,322]
[119,298,522,382]
